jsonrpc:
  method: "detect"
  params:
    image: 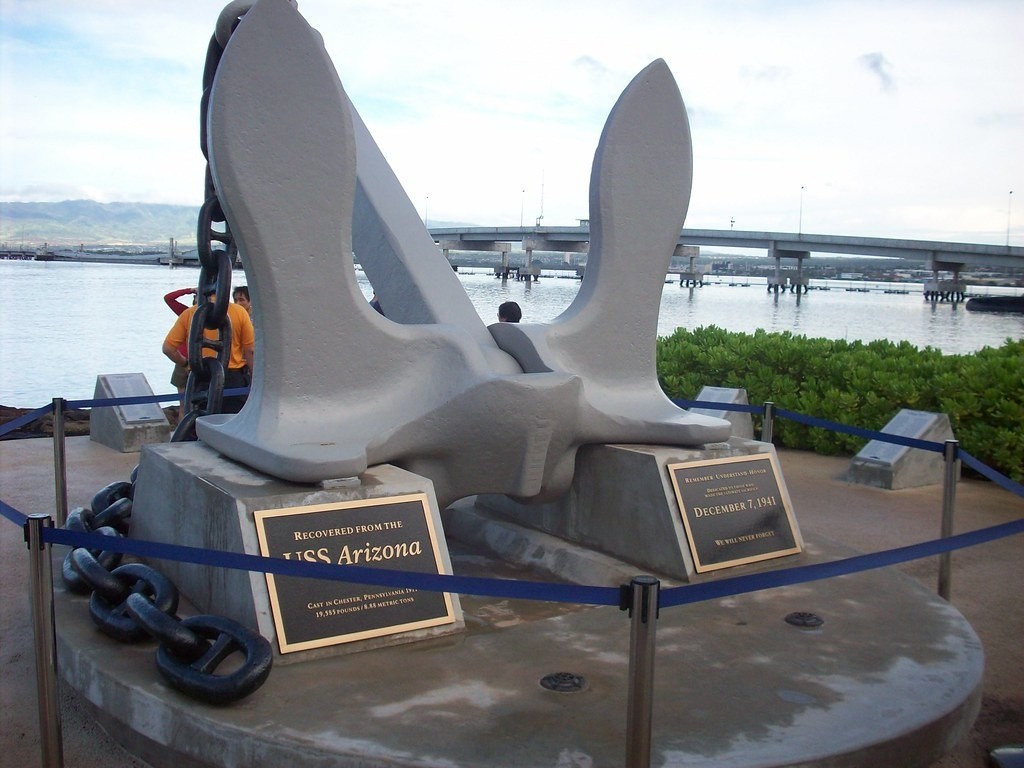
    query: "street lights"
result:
[799,186,804,233]
[1007,191,1012,246]
[521,190,525,226]
[425,197,429,228]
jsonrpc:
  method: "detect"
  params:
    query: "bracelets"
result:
[182,360,188,367]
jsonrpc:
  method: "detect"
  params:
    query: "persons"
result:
[162,286,253,425]
[368,291,384,316]
[498,302,522,323]
